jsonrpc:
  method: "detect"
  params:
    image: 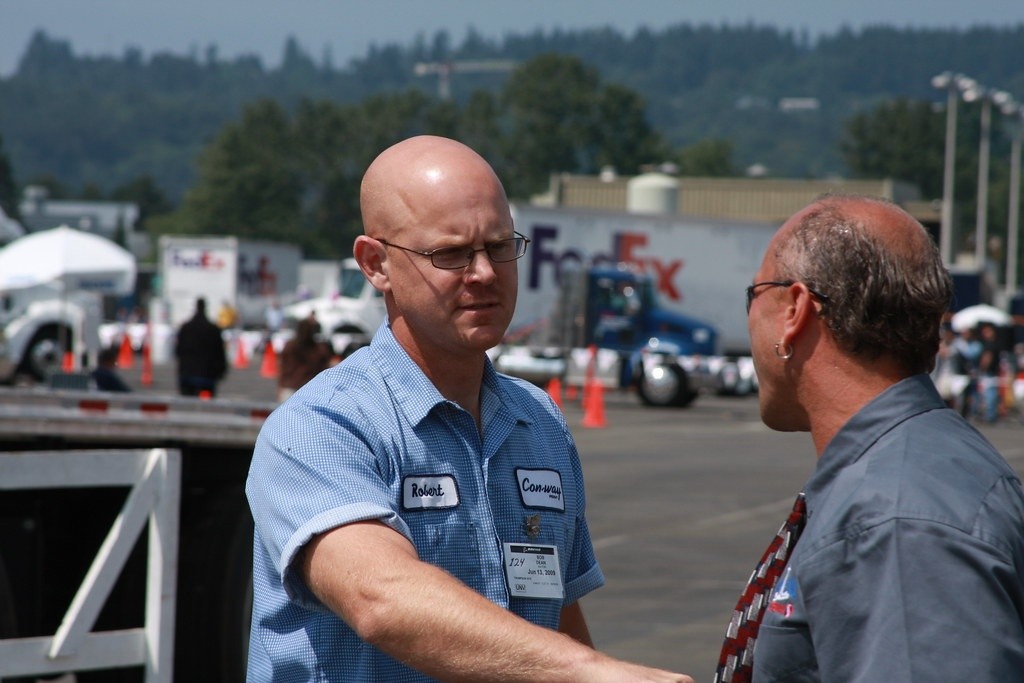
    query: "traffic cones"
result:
[115,336,135,370]
[546,378,564,414]
[579,381,611,432]
[62,349,75,375]
[234,343,250,370]
[259,337,279,380]
[141,346,153,386]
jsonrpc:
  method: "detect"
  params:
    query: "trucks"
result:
[498,259,715,408]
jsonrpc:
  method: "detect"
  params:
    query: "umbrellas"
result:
[0,223,136,353]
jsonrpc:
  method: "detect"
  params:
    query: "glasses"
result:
[746,280,829,315]
[375,231,531,270]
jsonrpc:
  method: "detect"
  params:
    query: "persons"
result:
[118,306,145,323]
[940,322,1000,422]
[174,299,228,398]
[260,298,285,331]
[245,134,695,683]
[308,310,321,334]
[279,320,330,401]
[609,280,632,307]
[713,197,1024,683]
[90,349,131,392]
[217,300,237,328]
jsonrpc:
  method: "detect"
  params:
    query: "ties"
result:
[713,490,806,683]
